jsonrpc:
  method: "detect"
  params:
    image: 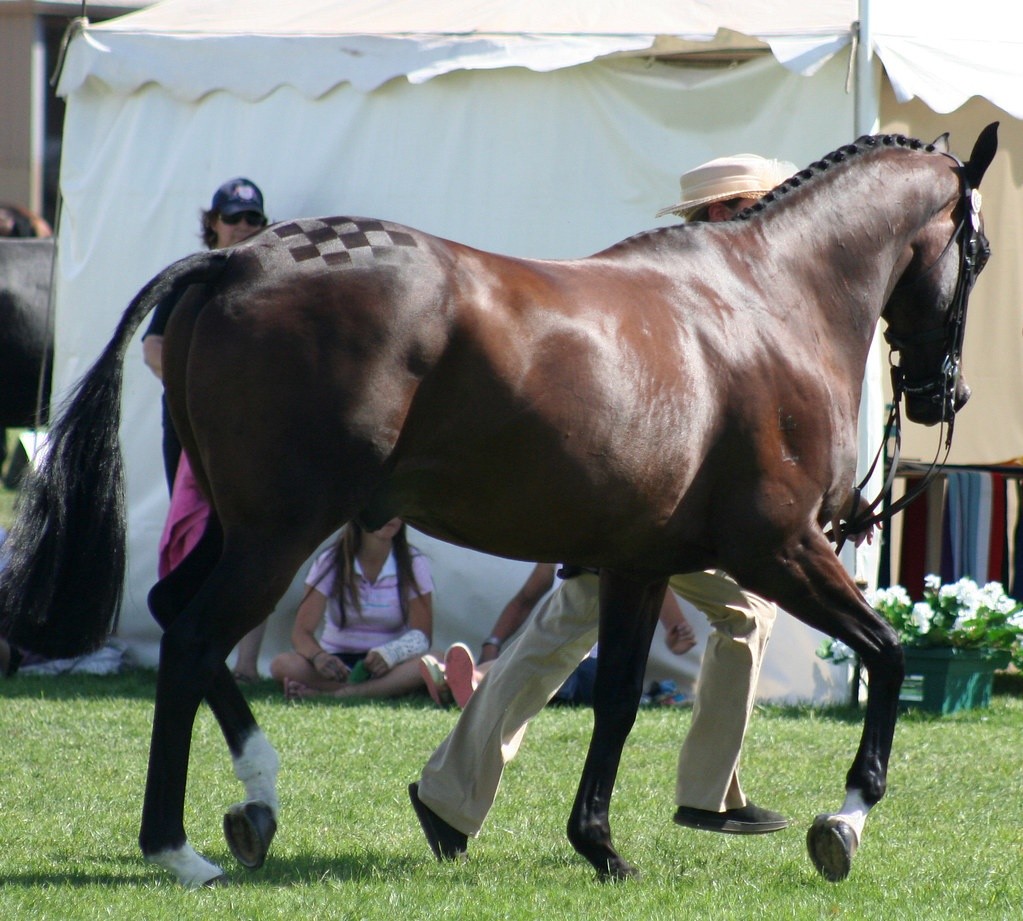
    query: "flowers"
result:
[815,574,1023,671]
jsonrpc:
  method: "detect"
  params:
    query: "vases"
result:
[899,646,1003,716]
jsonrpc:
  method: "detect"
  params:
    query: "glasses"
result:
[215,212,265,226]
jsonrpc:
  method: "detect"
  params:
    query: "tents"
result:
[12,0,1023,713]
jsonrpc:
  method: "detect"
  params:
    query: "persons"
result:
[407,151,883,866]
[418,563,698,712]
[269,514,447,706]
[141,177,270,692]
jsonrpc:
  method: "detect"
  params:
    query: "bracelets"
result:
[369,628,430,671]
[307,648,326,662]
[480,637,501,651]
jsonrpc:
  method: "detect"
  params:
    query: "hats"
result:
[210,179,263,216]
[655,153,800,217]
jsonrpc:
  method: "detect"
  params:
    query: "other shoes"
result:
[283,677,322,701]
[409,780,468,869]
[674,797,787,833]
[230,672,266,692]
[420,654,456,710]
[444,641,478,711]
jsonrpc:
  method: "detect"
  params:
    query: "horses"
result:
[1,115,1001,887]
